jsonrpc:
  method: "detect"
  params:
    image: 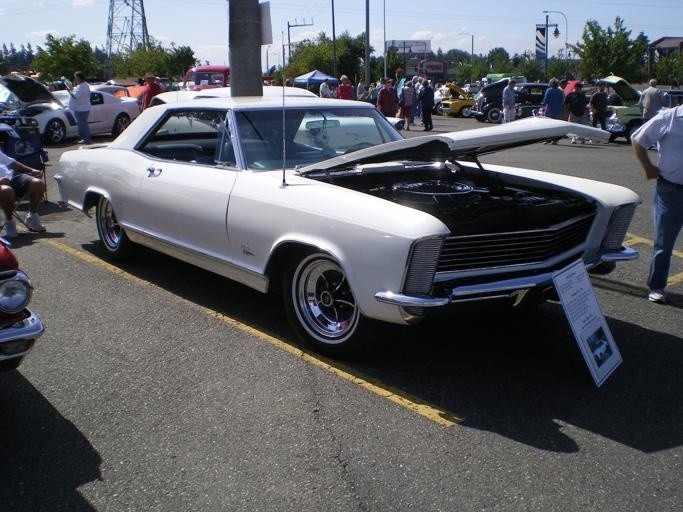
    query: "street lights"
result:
[545,15,560,84]
[396,40,413,76]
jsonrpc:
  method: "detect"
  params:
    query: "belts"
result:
[657,175,683,189]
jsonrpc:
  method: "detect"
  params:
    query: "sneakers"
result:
[649,288,664,302]
[5,219,18,238]
[77,139,87,144]
[24,214,45,232]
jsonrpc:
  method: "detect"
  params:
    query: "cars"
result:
[54,33,643,355]
[149,86,406,153]
[0,236,45,372]
[440,82,475,118]
[432,84,467,115]
[589,74,683,145]
[0,71,179,145]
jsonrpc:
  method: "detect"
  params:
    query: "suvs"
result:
[471,78,551,122]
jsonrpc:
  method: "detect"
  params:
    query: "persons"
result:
[543,78,564,145]
[60,75,66,89]
[502,80,518,122]
[564,84,587,144]
[638,79,665,150]
[0,151,46,238]
[284,79,290,86]
[319,67,488,131]
[67,71,92,144]
[630,105,682,302]
[264,79,278,86]
[560,79,573,138]
[129,75,165,111]
[589,81,608,143]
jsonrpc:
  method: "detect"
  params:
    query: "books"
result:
[64,79,72,90]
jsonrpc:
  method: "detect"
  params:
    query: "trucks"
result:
[181,64,229,90]
